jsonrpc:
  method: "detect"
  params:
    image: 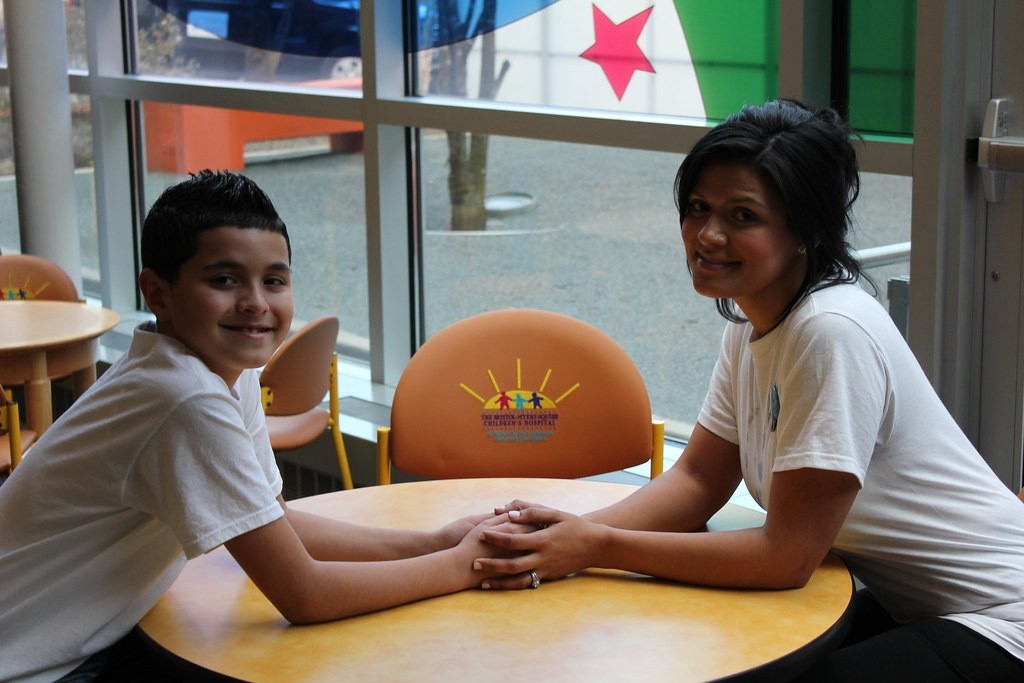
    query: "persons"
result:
[0,170,545,683]
[474,97,1024,683]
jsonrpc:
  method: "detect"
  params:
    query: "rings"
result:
[529,569,540,588]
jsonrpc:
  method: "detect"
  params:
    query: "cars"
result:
[161,20,467,83]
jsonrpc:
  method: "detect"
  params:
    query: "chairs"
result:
[377,309,664,485]
[2,255,78,302]
[0,387,36,473]
[260,316,353,491]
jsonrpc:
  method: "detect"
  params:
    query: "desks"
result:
[136,477,855,683]
[0,301,120,441]
